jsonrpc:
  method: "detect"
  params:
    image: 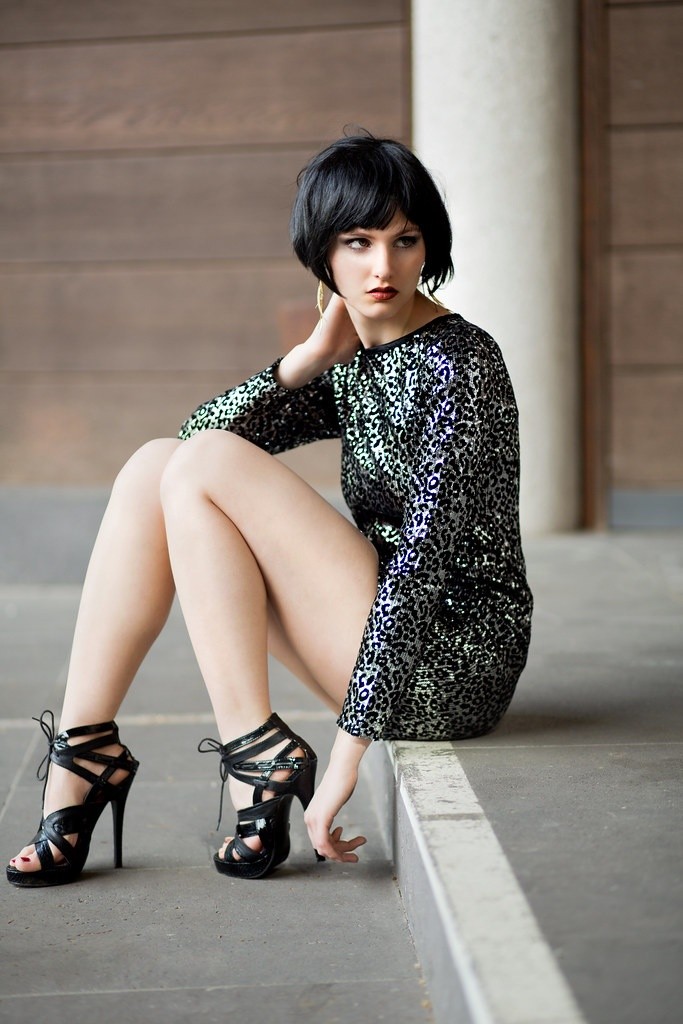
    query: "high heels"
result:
[197,712,326,879]
[6,710,140,888]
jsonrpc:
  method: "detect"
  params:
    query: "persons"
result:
[7,133,534,890]
